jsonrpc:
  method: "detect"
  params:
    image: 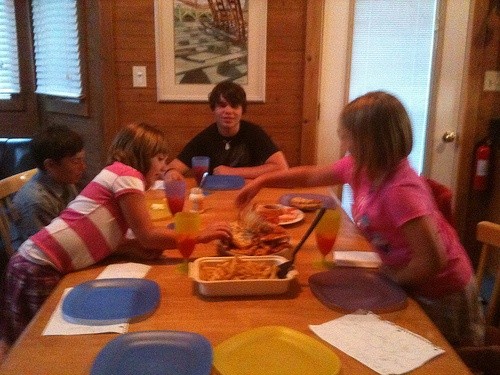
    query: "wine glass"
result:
[315,208,342,271]
[164,180,185,230]
[173,211,202,275]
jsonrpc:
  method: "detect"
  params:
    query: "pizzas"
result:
[219,219,291,254]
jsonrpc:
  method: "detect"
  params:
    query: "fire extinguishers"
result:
[472,136,494,193]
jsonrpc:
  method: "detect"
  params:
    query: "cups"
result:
[192,156,209,184]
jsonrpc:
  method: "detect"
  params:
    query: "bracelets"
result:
[163,167,176,178]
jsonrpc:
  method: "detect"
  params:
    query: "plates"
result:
[308,268,408,313]
[206,174,245,190]
[61,277,160,325]
[277,206,304,225]
[145,197,173,221]
[90,330,213,375]
[211,325,342,375]
[279,193,337,210]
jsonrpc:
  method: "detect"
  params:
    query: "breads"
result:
[289,196,322,210]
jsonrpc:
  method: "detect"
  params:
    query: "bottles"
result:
[188,187,204,213]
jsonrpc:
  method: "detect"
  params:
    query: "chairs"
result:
[457,221,500,362]
[0,168,38,258]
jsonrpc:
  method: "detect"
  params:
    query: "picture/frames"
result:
[154,0,268,103]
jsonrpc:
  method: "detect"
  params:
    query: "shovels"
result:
[276,205,327,279]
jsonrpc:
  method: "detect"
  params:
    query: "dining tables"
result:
[0,179,470,375]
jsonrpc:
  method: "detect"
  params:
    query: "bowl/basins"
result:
[188,255,298,297]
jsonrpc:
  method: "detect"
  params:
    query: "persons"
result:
[7,125,87,253]
[233,91,484,364]
[162,81,289,181]
[5,121,233,344]
